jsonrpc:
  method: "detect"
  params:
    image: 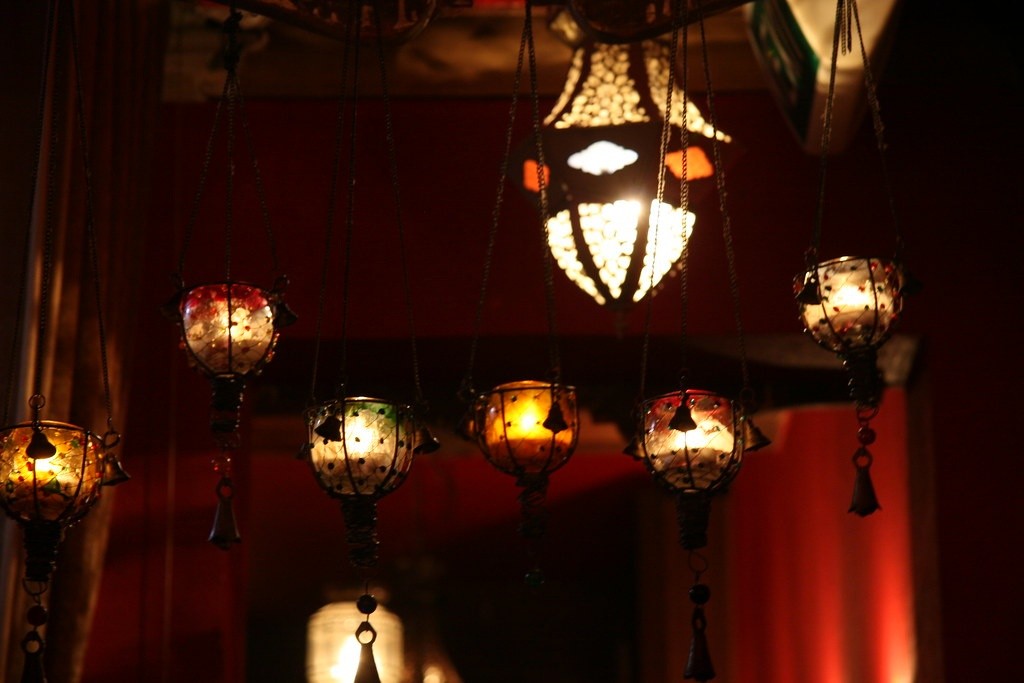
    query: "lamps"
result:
[1,0,913,683]
